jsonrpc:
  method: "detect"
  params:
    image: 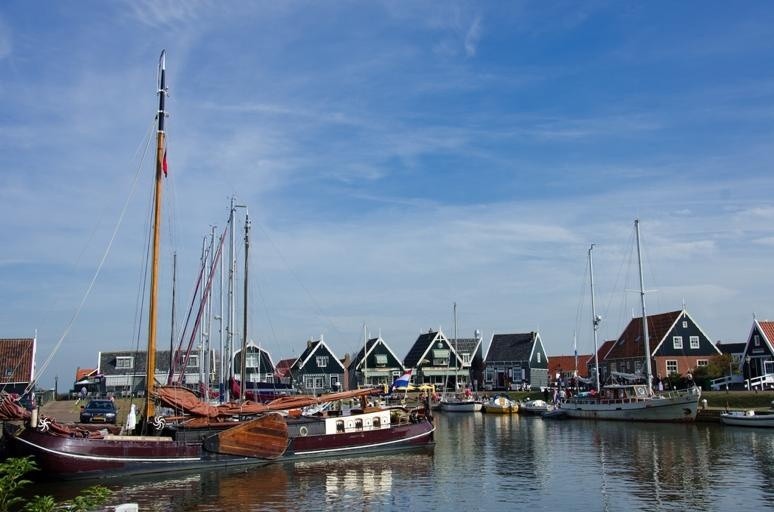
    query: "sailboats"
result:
[547,427,710,506]
[9,48,436,479]
[553,218,701,423]
[164,192,335,418]
[438,299,483,412]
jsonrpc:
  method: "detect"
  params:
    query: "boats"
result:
[720,410,774,428]
[33,452,434,509]
[484,397,518,413]
[520,399,555,413]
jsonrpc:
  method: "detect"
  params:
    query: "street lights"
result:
[53,375,60,400]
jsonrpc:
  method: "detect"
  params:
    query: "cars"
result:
[79,398,118,424]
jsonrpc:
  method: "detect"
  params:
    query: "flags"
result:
[393,369,411,389]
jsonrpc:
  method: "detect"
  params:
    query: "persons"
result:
[421,370,695,404]
[108,393,115,402]
